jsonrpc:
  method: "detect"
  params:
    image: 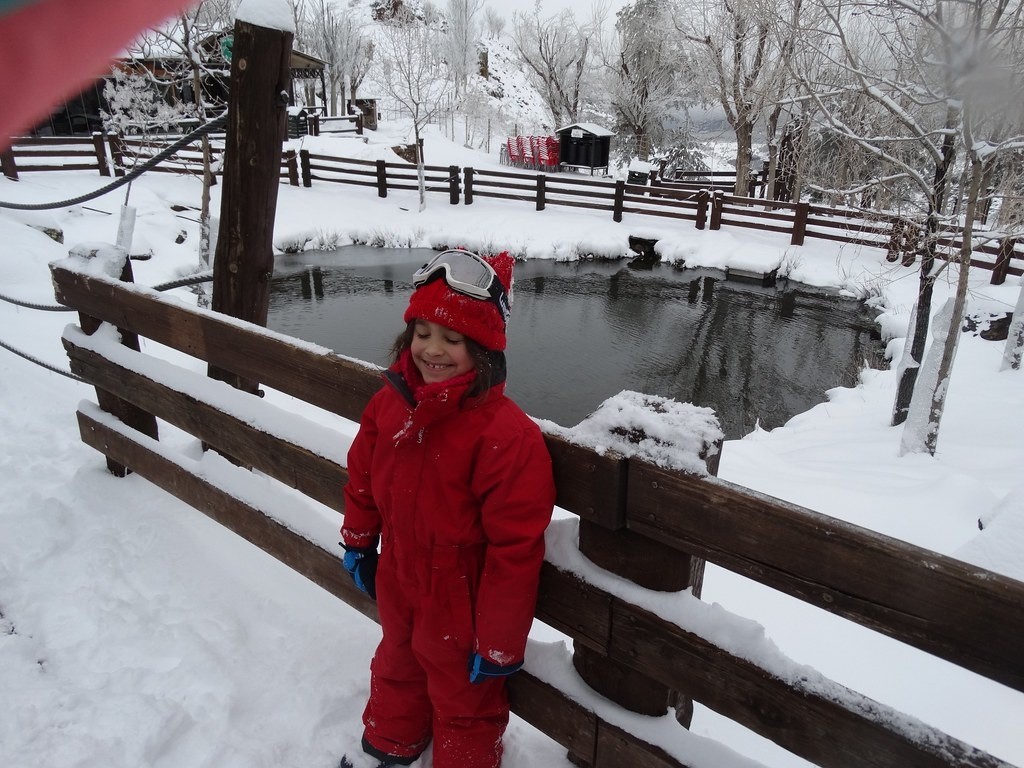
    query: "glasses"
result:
[413,249,497,301]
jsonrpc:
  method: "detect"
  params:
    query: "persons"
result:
[338,247,556,768]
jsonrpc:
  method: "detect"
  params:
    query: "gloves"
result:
[466,653,525,684]
[338,535,378,603]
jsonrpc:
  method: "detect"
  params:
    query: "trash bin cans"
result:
[625,170,648,195]
[347,98,380,131]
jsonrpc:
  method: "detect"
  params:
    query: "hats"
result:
[403,248,515,351]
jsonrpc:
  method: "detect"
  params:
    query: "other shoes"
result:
[339,733,422,768]
